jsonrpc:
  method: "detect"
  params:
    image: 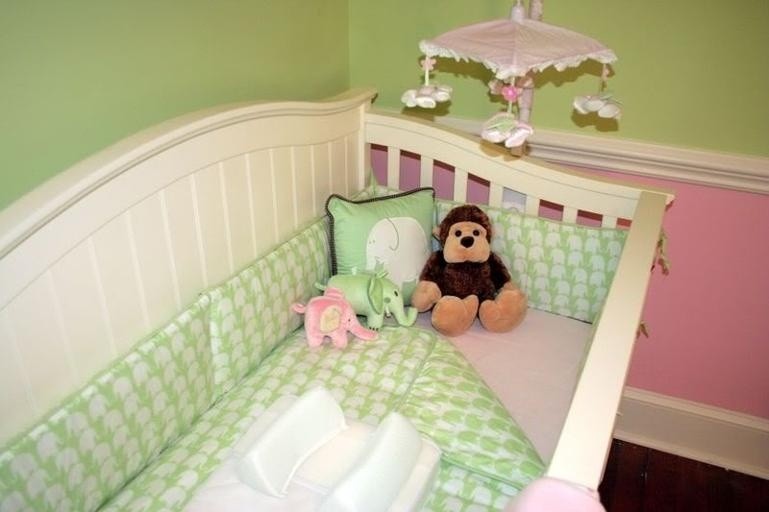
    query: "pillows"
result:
[326,188,437,306]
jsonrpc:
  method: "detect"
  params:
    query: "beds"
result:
[1,85,676,512]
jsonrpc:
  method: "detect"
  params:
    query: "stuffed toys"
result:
[314,268,417,331]
[291,287,378,351]
[410,204,527,337]
